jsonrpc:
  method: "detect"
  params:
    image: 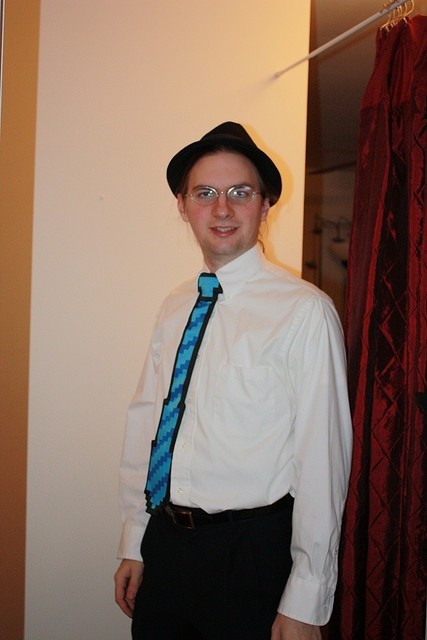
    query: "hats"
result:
[167,122,282,207]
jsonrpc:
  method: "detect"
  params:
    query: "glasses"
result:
[183,184,263,206]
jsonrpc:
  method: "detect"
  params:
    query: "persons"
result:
[111,120,356,638]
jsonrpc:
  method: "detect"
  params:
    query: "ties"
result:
[144,274,223,515]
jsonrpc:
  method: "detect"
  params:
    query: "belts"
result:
[160,496,288,532]
